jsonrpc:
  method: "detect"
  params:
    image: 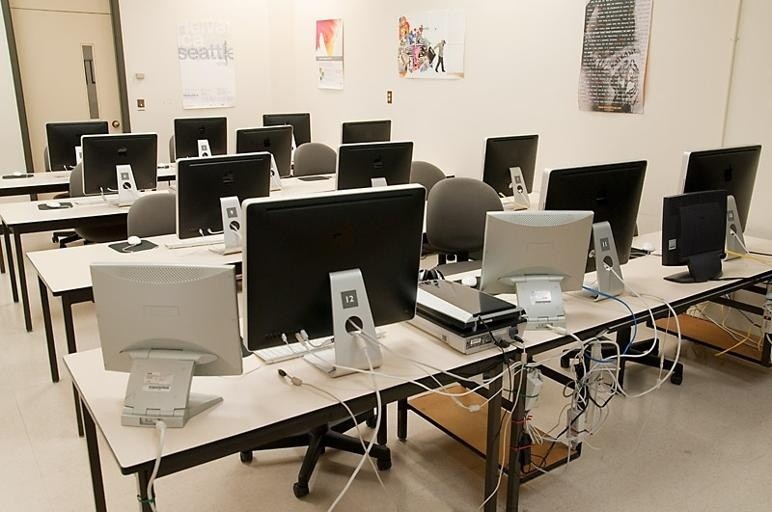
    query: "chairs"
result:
[127,193,176,237]
[408,161,449,198]
[293,142,337,176]
[52,163,118,244]
[43,143,83,172]
[426,178,504,277]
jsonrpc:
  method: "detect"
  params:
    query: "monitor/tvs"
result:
[89,264,243,428]
[81,132,158,207]
[342,120,391,144]
[176,152,271,254]
[235,125,292,191]
[263,113,311,165]
[538,158,648,302]
[676,143,762,262]
[335,140,413,190]
[174,117,227,161]
[241,183,427,378]
[45,121,109,172]
[662,189,727,283]
[480,134,539,211]
[480,211,594,330]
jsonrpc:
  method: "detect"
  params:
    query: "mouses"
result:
[127,235,142,245]
[14,171,22,176]
[46,201,61,208]
[644,242,655,251]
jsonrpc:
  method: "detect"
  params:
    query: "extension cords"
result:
[762,293,772,334]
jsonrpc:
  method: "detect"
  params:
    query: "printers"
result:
[408,279,527,355]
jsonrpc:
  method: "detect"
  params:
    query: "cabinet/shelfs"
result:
[631,231,772,368]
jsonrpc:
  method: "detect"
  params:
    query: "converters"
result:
[524,373,543,412]
[567,408,585,444]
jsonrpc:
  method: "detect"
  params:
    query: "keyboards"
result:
[74,194,118,205]
[254,326,388,365]
[165,233,225,249]
[651,248,662,256]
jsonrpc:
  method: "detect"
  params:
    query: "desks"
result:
[263,169,338,196]
[1,163,179,276]
[0,189,206,330]
[501,192,539,216]
[436,252,772,511]
[61,309,517,512]
[25,225,310,382]
